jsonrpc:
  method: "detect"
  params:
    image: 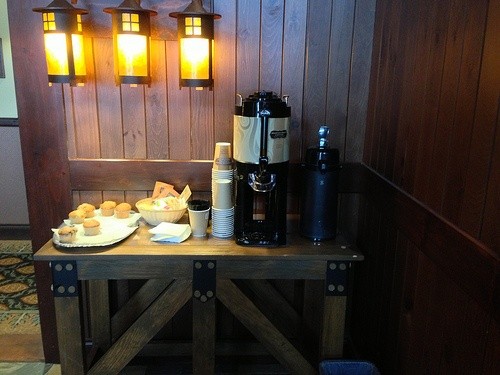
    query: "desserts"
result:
[57,200,132,242]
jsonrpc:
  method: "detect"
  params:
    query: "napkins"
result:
[51,223,130,243]
[63,210,141,226]
[148,222,192,243]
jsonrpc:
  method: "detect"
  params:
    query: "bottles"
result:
[303,125,339,242]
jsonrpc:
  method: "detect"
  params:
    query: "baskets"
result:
[135,196,188,226]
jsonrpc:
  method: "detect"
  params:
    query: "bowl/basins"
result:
[135,199,189,227]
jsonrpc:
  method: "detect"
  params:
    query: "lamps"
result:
[102,0,158,88]
[169,0,222,91]
[32,0,89,87]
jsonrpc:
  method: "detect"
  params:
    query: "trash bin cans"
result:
[319,360,378,375]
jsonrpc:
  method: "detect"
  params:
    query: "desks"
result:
[33,214,364,375]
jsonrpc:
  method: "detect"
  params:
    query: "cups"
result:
[187,207,210,238]
[209,142,236,239]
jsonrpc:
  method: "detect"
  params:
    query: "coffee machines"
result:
[234,91,291,248]
